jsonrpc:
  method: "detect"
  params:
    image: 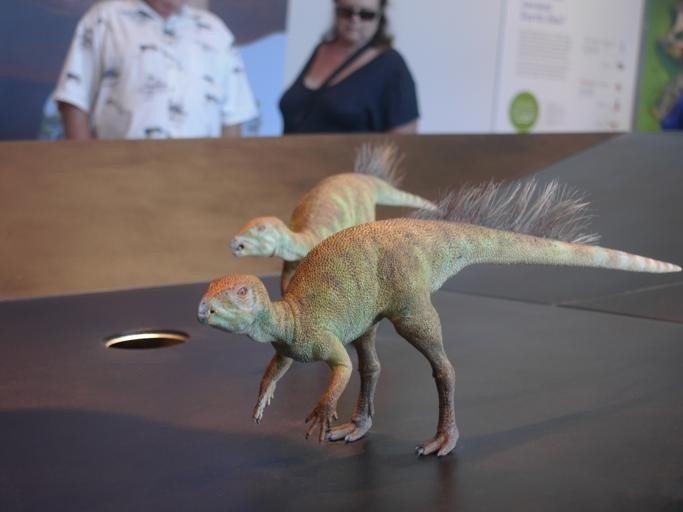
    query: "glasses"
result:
[336,6,381,21]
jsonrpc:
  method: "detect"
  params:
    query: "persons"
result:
[279,0,420,134]
[52,0,260,141]
